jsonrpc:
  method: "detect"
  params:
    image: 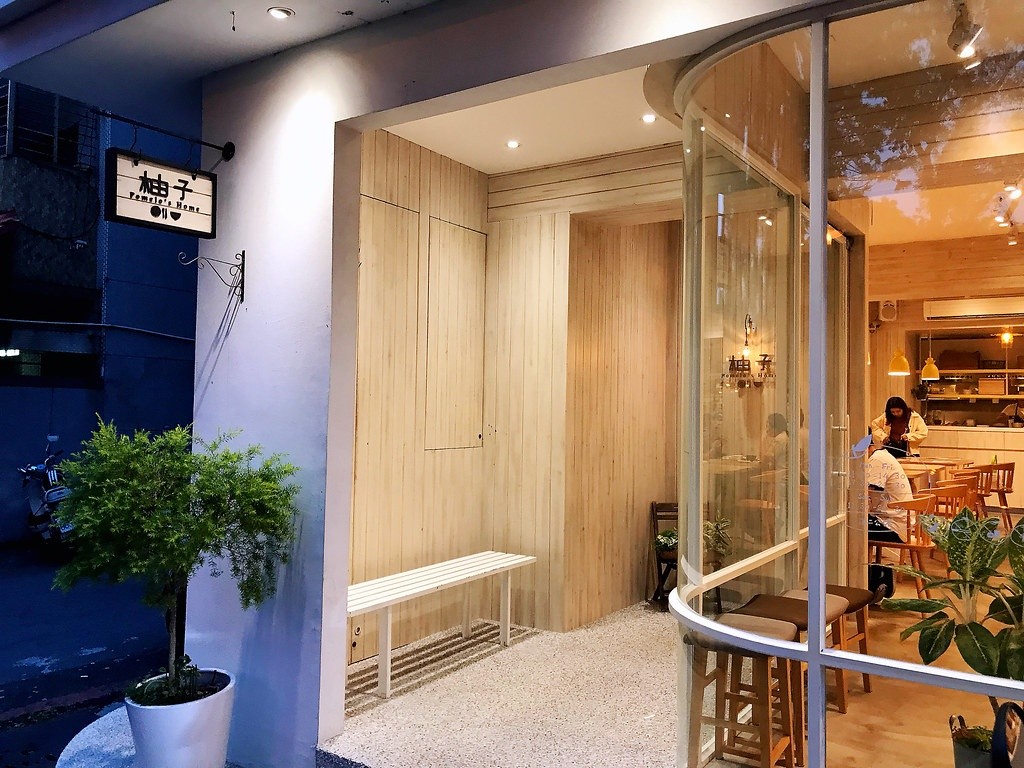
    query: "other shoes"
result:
[868,563,897,606]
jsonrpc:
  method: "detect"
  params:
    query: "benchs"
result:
[347,550,536,699]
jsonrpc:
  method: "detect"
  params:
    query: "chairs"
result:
[646,502,723,614]
[949,468,980,519]
[937,476,978,520]
[732,469,789,554]
[983,462,1015,535]
[918,484,967,558]
[964,465,994,520]
[869,494,936,621]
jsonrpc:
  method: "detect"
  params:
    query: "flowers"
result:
[653,530,678,552]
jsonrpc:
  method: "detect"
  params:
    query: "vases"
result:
[660,551,678,559]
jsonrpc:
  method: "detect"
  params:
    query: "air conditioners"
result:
[923,296,1024,322]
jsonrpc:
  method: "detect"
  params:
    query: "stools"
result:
[683,584,873,768]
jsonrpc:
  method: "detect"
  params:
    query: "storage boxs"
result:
[977,378,1005,395]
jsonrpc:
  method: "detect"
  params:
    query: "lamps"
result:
[920,330,940,380]
[739,312,758,357]
[888,300,910,376]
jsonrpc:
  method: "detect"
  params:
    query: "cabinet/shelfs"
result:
[915,368,1024,432]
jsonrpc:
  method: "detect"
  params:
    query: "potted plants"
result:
[703,514,731,562]
[50,419,303,768]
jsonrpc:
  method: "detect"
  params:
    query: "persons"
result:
[703,437,727,462]
[756,407,809,487]
[867,426,916,605]
[871,397,928,457]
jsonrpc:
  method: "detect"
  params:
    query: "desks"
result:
[797,483,846,582]
[703,456,760,522]
[892,457,974,494]
